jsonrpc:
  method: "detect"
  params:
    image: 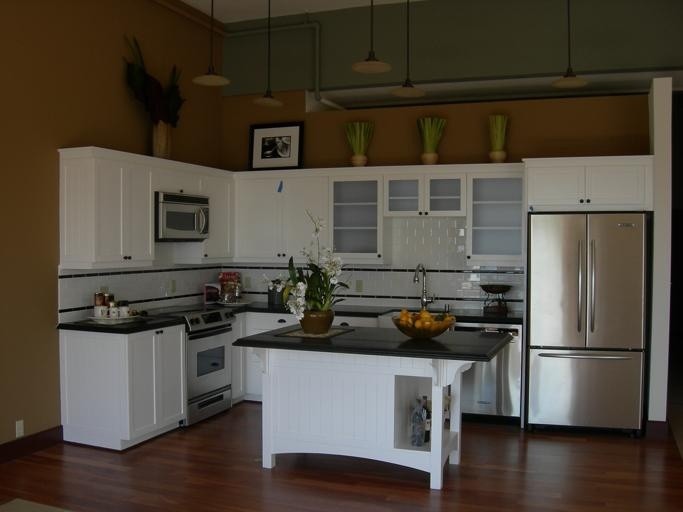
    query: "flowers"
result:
[263,201,352,318]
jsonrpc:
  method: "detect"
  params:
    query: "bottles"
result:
[409,399,424,446]
[218,272,241,302]
[266,277,287,306]
[422,395,430,442]
[93,291,129,318]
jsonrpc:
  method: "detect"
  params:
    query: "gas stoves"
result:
[159,307,237,332]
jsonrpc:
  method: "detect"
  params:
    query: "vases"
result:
[299,309,336,336]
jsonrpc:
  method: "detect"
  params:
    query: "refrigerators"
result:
[527,213,645,429]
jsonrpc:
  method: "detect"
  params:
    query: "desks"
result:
[232,319,514,491]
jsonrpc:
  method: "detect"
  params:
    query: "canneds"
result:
[95,293,116,315]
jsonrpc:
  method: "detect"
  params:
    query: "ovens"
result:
[187,327,231,425]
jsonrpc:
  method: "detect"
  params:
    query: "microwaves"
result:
[158,192,209,240]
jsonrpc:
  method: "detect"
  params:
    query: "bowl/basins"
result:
[390,316,455,340]
[479,284,511,294]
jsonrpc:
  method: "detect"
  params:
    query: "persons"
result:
[274,137,290,158]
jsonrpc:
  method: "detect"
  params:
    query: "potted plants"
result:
[485,113,509,162]
[122,31,185,161]
[416,115,446,166]
[338,122,375,165]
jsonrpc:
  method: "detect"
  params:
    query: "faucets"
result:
[413,263,435,308]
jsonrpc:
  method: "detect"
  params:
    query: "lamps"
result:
[192,1,231,88]
[389,0,422,100]
[348,0,393,77]
[253,1,285,108]
[550,1,587,91]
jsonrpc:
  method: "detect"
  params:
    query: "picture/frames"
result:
[248,119,303,171]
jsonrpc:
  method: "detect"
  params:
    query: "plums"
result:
[399,309,455,330]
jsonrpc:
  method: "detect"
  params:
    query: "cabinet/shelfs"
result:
[241,310,299,404]
[374,162,466,223]
[228,167,328,273]
[328,312,394,328]
[450,322,525,425]
[461,162,528,277]
[171,163,234,268]
[523,149,656,215]
[60,322,186,451]
[148,156,202,196]
[56,143,157,273]
[324,165,393,271]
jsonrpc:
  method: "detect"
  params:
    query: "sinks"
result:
[378,311,443,329]
